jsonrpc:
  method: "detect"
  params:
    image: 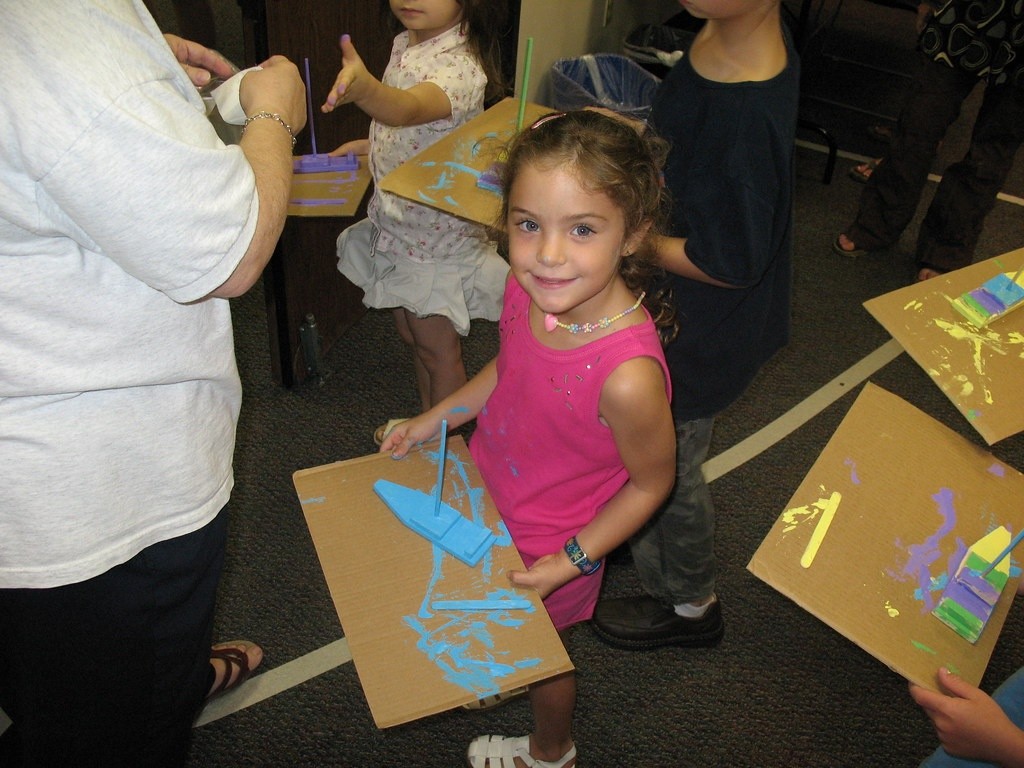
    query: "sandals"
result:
[469,735,577,768]
[463,686,529,709]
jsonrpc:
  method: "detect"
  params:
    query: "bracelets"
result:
[561,537,601,576]
[236,110,299,148]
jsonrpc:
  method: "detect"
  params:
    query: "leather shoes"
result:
[593,592,725,648]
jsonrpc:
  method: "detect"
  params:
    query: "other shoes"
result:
[208,639,263,701]
[850,157,884,183]
[374,419,408,445]
[833,233,897,257]
[867,123,944,159]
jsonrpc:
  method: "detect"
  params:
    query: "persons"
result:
[832,0,1024,282]
[378,109,675,768]
[904,667,1024,768]
[0,0,308,768]
[321,0,794,647]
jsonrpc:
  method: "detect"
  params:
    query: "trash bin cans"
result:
[622,22,698,80]
[551,53,662,119]
